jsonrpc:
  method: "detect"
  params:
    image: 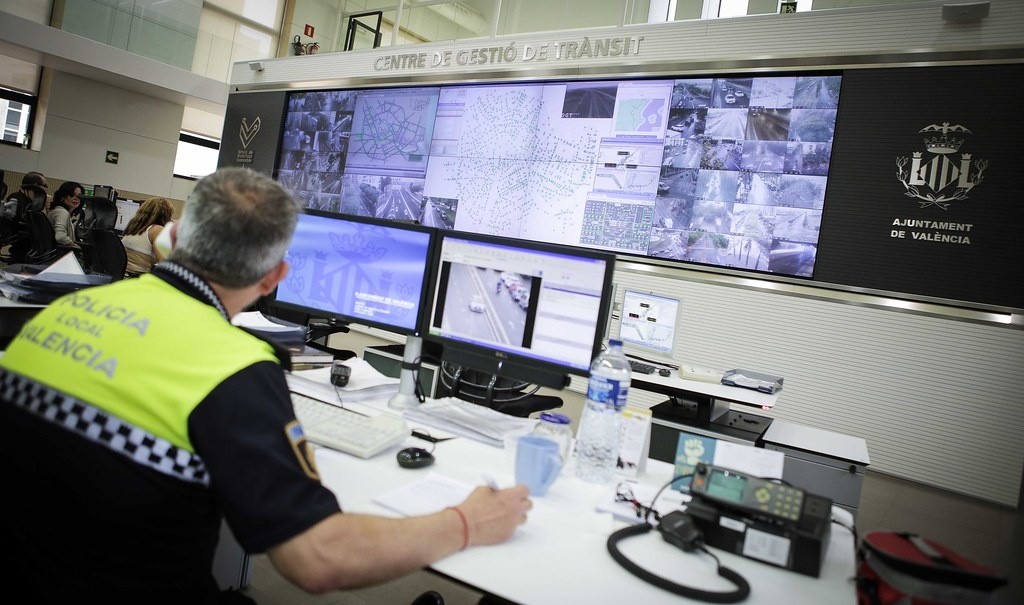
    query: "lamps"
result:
[249,62,265,72]
[942,1,991,24]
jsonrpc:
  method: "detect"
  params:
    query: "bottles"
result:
[572,339,632,483]
[534,412,574,468]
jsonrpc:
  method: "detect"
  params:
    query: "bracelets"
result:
[445,506,469,550]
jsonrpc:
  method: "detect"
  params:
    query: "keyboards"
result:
[288,391,409,459]
[627,360,655,375]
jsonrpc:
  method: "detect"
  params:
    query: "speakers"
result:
[249,62,265,71]
[941,2,991,21]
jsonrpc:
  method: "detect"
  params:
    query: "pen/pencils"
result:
[481,474,499,495]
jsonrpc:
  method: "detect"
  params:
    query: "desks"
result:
[239,360,860,605]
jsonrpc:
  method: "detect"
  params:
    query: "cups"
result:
[514,436,564,497]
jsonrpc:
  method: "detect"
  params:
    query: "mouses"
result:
[659,369,671,377]
[397,446,435,469]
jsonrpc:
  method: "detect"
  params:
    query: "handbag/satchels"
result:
[859,530,1011,605]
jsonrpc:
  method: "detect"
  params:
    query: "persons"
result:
[0,172,177,280]
[0,166,532,605]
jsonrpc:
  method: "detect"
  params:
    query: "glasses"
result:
[38,184,49,190]
[70,193,84,200]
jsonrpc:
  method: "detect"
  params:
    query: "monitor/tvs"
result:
[421,228,617,416]
[263,208,438,357]
[618,288,681,360]
[93,185,115,202]
[114,196,142,235]
